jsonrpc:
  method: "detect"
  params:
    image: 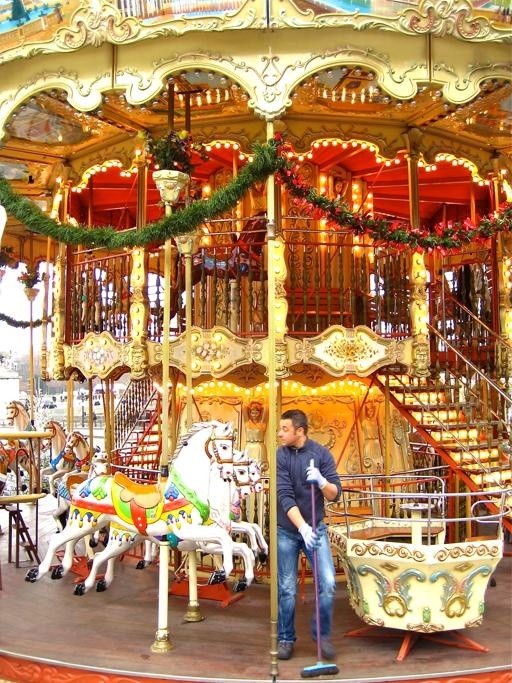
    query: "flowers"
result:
[148,132,210,174]
[273,133,512,257]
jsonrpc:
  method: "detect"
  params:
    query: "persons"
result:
[275,408,343,662]
[243,400,270,473]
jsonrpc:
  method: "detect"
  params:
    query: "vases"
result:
[151,170,190,207]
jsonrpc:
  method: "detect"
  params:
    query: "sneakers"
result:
[273,640,296,662]
[312,632,339,658]
